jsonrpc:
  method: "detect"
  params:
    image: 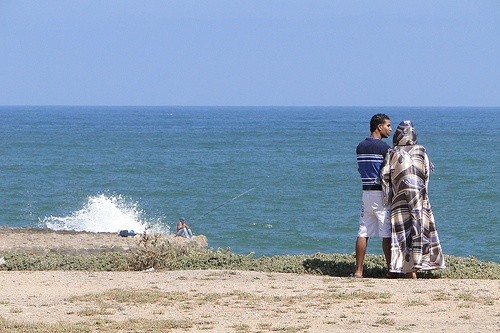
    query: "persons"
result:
[353,113,398,278]
[176,218,193,238]
[378,120,446,279]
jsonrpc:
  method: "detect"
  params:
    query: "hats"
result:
[180,217,185,220]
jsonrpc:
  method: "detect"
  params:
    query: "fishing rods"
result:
[190,184,262,226]
